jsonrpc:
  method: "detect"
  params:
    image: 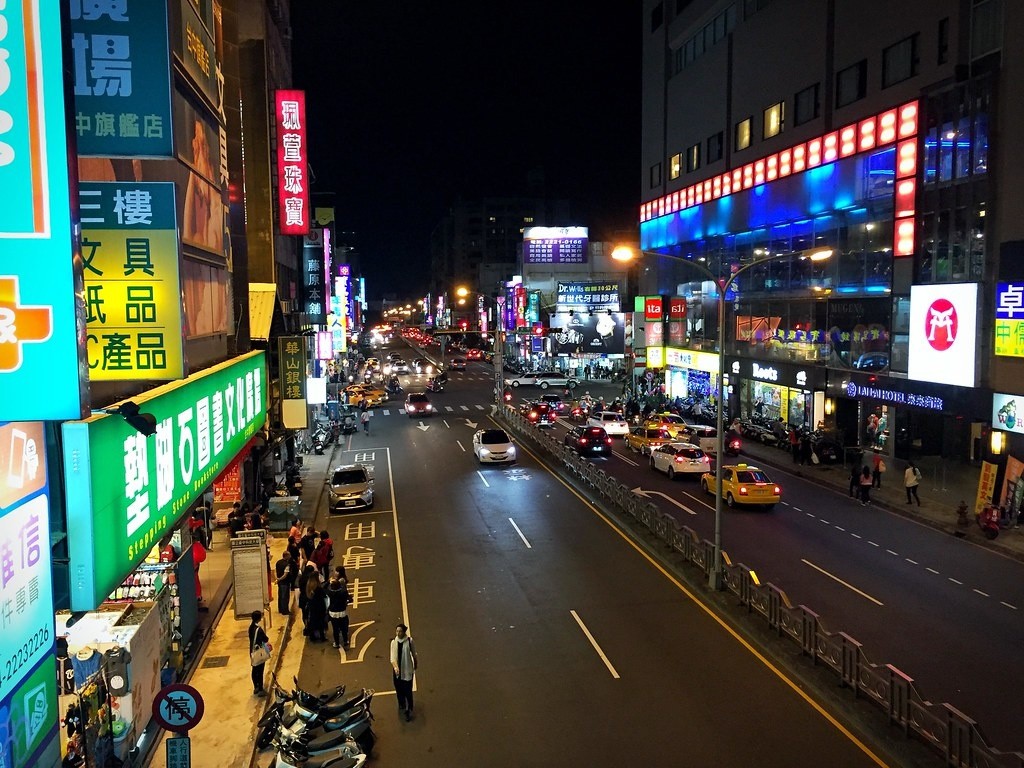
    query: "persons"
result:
[428,368,448,392]
[867,413,890,450]
[275,515,351,649]
[228,502,248,538]
[204,501,216,552]
[250,514,262,529]
[788,426,814,467]
[265,540,274,602]
[755,397,770,416]
[771,417,787,445]
[389,623,418,721]
[192,530,206,602]
[901,462,922,507]
[294,361,404,452]
[566,376,705,427]
[249,611,269,697]
[848,449,882,506]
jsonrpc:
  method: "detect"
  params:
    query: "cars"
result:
[503,369,844,467]
[404,392,433,418]
[337,320,483,418]
[700,463,783,510]
[326,463,375,515]
[564,425,613,459]
[472,428,517,467]
[649,442,712,482]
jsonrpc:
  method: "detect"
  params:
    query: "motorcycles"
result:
[256,670,378,768]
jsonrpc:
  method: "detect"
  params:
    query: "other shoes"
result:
[917,500,920,507]
[254,688,258,694]
[405,710,413,722]
[332,642,339,648]
[906,501,912,505]
[400,707,405,714]
[875,485,881,491]
[282,612,292,616]
[344,641,349,646]
[258,690,269,697]
[861,503,866,507]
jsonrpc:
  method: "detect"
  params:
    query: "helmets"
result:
[573,391,620,400]
[691,428,697,435]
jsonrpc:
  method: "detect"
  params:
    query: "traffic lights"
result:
[534,326,544,335]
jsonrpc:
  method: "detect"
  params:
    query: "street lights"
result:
[611,241,835,592]
[457,287,541,419]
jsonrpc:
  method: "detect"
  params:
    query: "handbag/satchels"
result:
[916,468,922,480]
[327,546,334,561]
[811,453,819,464]
[251,645,270,666]
[879,460,886,472]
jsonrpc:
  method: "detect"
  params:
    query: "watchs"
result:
[596,314,616,347]
[552,326,584,345]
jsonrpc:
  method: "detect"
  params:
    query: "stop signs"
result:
[152,683,205,734]
